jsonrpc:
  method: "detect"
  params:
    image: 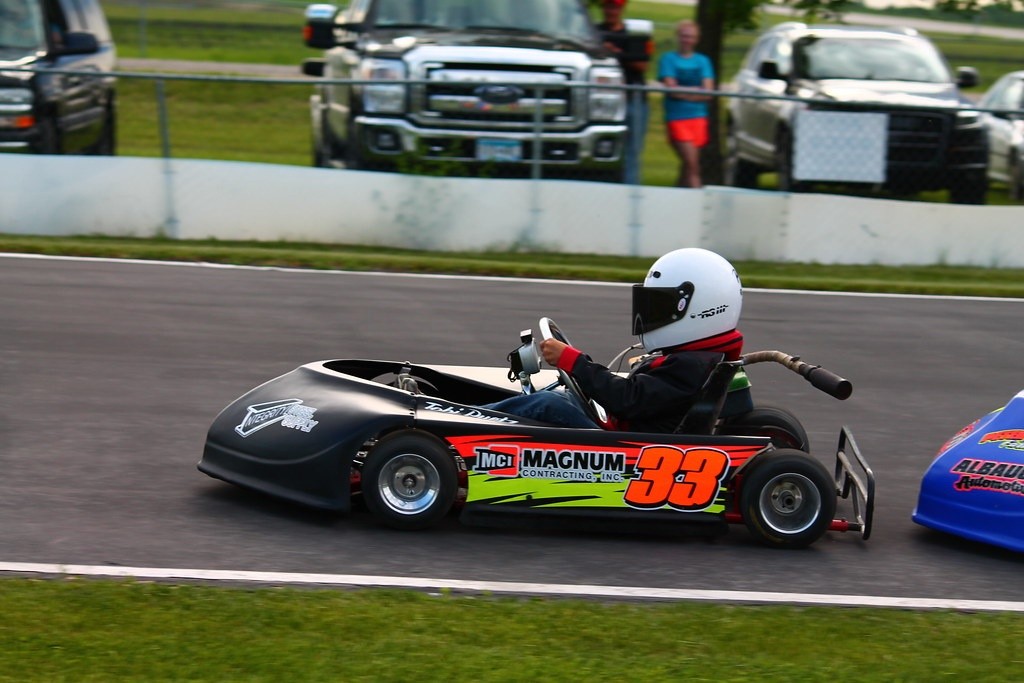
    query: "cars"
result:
[972,70,1024,192]
[0,0,117,154]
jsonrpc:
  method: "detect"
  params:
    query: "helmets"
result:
[630,250,743,354]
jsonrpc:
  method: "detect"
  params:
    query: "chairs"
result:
[673,359,743,435]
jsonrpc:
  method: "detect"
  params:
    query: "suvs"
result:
[719,22,990,204]
[302,0,653,180]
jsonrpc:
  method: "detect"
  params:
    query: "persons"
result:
[592,0,654,186]
[654,19,716,189]
[476,247,743,435]
